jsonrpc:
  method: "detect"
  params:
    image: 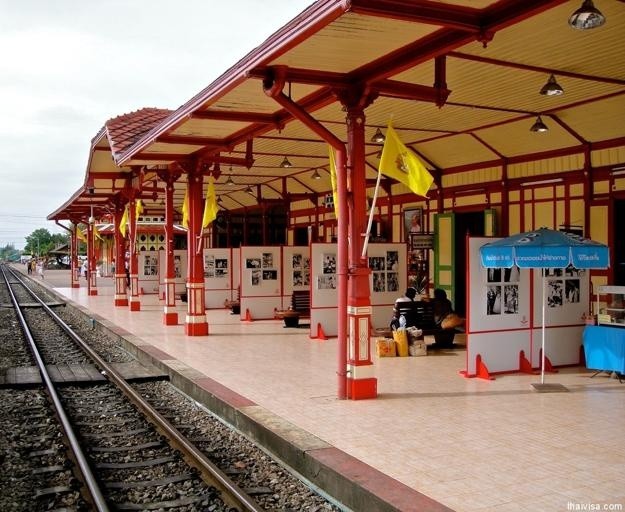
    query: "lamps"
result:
[225,176,234,184]
[539,73,564,96]
[279,156,292,168]
[529,115,549,132]
[216,196,223,203]
[244,185,253,193]
[311,169,321,179]
[568,0,606,29]
[376,146,383,159]
[370,128,385,143]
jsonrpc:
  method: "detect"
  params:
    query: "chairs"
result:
[276,290,310,327]
[391,301,455,346]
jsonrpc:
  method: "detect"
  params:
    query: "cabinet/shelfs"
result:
[597,286,625,326]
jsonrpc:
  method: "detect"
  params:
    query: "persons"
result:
[144,251,398,294]
[430,288,457,349]
[410,214,421,231]
[485,261,580,316]
[607,292,625,322]
[390,287,418,331]
[21,243,131,288]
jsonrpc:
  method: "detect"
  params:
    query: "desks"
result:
[582,325,625,384]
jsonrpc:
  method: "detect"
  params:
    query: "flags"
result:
[328,144,338,219]
[93,222,105,243]
[201,177,219,228]
[135,199,143,221]
[181,185,191,229]
[379,123,434,197]
[118,207,128,237]
[76,227,87,244]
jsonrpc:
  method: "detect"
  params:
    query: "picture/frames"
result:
[402,206,424,252]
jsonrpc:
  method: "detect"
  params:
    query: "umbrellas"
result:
[479,227,610,393]
